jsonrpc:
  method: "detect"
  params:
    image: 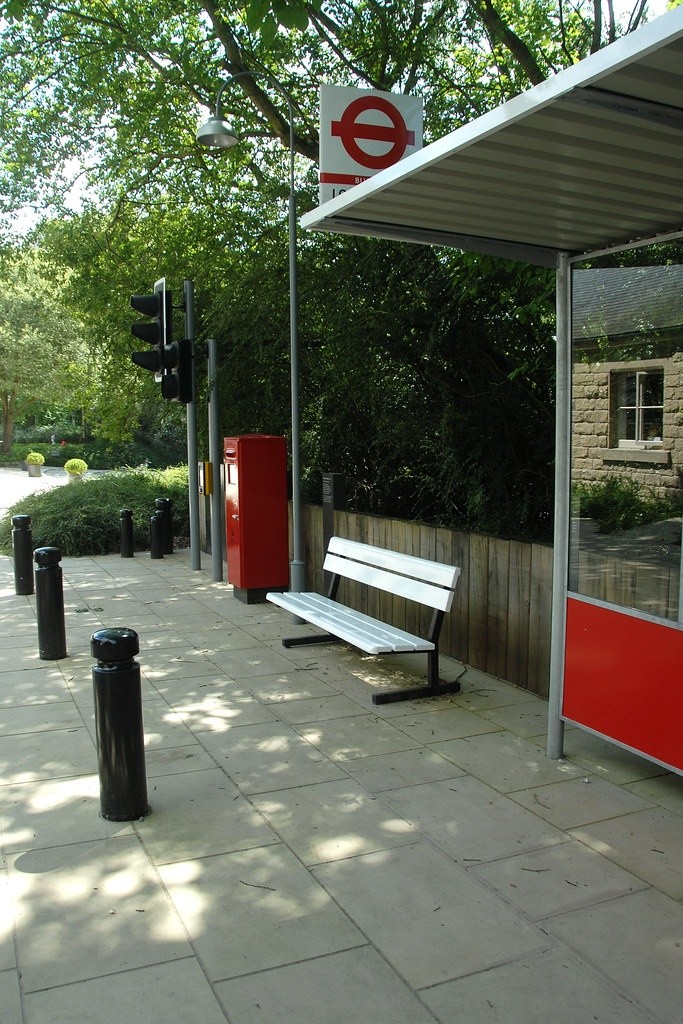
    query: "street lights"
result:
[193,66,307,600]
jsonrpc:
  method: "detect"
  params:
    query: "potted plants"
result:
[25,451,45,478]
[18,447,34,471]
[64,458,88,485]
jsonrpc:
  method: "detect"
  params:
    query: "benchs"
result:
[267,535,463,706]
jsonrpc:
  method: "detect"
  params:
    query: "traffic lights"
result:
[160,338,194,406]
[130,277,171,383]
